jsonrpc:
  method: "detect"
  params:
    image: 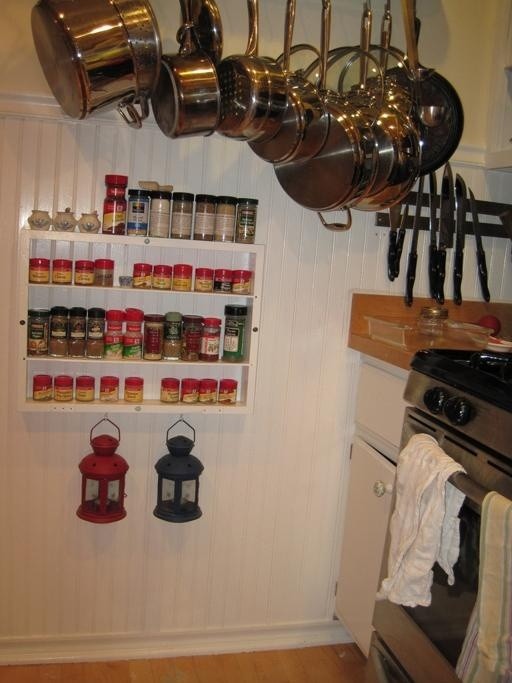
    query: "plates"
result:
[363,315,494,351]
[472,336,512,353]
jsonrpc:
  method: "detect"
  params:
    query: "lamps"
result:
[153,419,203,522]
[76,417,128,524]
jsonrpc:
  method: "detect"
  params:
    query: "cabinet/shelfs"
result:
[16,229,265,415]
[336,334,412,660]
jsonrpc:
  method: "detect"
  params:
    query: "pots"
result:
[340,0,422,212]
[32,0,143,129]
[113,0,163,121]
[273,0,379,231]
[152,0,222,139]
[180,1,223,67]
[215,0,288,147]
[247,1,329,166]
[369,0,423,134]
[385,0,465,178]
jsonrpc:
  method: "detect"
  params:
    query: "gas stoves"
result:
[403,347,512,462]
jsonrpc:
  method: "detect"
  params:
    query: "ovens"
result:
[371,416,512,683]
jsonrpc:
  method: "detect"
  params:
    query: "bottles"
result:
[143,313,165,360]
[69,307,87,331]
[232,270,252,295]
[170,191,195,239]
[221,304,248,362]
[181,315,203,361]
[28,308,50,358]
[29,258,51,284]
[99,375,119,402]
[68,332,85,357]
[94,259,114,288]
[123,308,144,359]
[101,174,128,235]
[74,260,94,286]
[127,189,149,236]
[194,268,214,293]
[52,260,73,285]
[193,194,216,241]
[180,378,199,403]
[417,306,448,349]
[87,307,105,332]
[172,264,193,291]
[235,197,259,244]
[199,318,222,362]
[124,377,144,402]
[132,263,153,289]
[32,374,52,401]
[49,306,69,330]
[213,269,232,294]
[151,265,172,291]
[165,337,180,360]
[214,195,237,242]
[49,330,68,358]
[165,311,182,337]
[76,375,95,401]
[217,379,238,405]
[160,378,180,403]
[86,332,103,358]
[104,309,125,360]
[53,375,73,401]
[199,379,218,404]
[148,190,173,238]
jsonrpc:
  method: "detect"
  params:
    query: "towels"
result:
[376,432,469,608]
[455,491,511,683]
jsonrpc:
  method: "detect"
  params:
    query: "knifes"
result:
[454,172,467,306]
[434,161,456,305]
[468,186,491,302]
[429,171,440,300]
[404,175,425,308]
[388,199,402,277]
[387,202,409,282]
